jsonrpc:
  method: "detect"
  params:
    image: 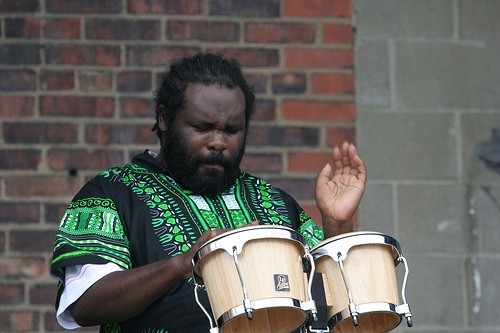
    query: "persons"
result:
[47,52,368,333]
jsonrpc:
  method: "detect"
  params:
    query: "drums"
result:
[308,231,412,333]
[191,225,318,333]
